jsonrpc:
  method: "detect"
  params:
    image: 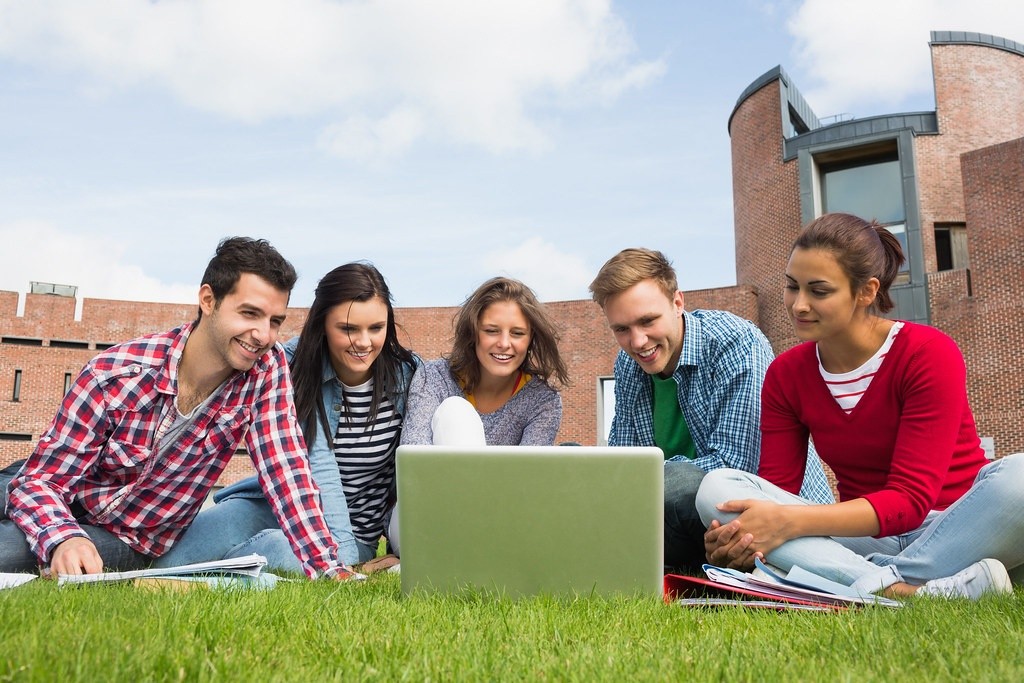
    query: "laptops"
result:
[393,445,666,607]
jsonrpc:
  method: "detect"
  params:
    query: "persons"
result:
[0,234,368,584]
[152,263,418,577]
[557,247,836,573]
[694,213,1024,601]
[381,276,577,559]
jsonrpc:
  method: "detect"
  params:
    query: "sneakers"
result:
[915,557,1016,604]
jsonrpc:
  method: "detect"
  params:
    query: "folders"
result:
[663,574,863,615]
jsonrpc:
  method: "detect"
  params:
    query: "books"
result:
[56,553,267,586]
[702,562,902,609]
